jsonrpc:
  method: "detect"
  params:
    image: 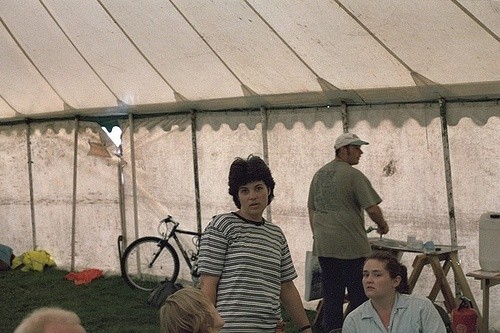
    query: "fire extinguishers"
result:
[451,295,478,333]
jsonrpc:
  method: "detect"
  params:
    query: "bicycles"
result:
[122,215,206,292]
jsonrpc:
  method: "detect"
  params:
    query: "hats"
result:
[334,133,369,150]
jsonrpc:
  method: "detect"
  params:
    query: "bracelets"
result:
[297,325,311,332]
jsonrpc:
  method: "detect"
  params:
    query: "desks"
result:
[312,238,484,333]
[466,270,500,333]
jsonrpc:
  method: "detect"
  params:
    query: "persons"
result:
[160,286,225,333]
[198,154,312,333]
[13,307,87,333]
[307,132,389,333]
[342,251,447,333]
